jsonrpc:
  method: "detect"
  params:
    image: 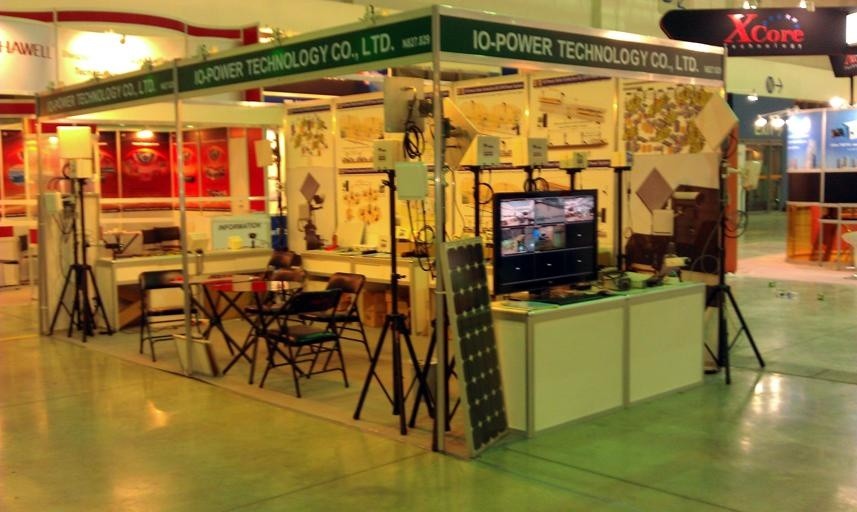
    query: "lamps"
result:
[747,95,803,128]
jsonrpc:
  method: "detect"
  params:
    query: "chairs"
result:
[259,289,349,398]
[138,270,203,363]
[294,272,374,370]
[243,254,304,348]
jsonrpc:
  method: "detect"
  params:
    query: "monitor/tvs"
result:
[492,188,604,306]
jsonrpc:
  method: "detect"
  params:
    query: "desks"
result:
[205,280,327,384]
[198,249,274,279]
[489,283,706,438]
[97,254,198,334]
[301,249,431,336]
[176,274,258,354]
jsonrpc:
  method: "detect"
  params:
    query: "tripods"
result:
[353,173,433,434]
[683,160,766,385]
[48,207,96,336]
[67,185,113,343]
[409,190,462,431]
